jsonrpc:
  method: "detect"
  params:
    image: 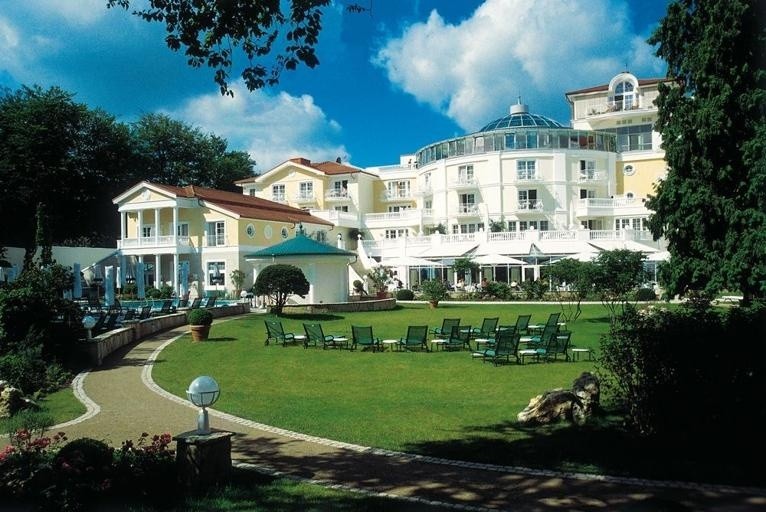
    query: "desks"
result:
[381,340,398,352]
[333,338,348,349]
[294,335,307,347]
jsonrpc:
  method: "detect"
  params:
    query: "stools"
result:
[570,348,594,362]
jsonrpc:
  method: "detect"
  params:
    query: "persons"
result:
[510,280,519,291]
[652,282,661,295]
[80,309,96,330]
[482,277,489,288]
[187,282,200,307]
[411,275,479,292]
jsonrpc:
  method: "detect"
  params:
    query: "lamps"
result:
[185,375,220,436]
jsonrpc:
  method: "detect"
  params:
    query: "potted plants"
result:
[188,307,212,342]
[367,265,392,299]
[420,278,450,309]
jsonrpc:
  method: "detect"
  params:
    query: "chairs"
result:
[302,323,346,349]
[350,325,383,354]
[428,314,574,366]
[265,320,296,348]
[399,325,429,351]
[80,296,216,338]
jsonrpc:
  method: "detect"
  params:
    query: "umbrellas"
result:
[211,262,223,308]
[546,236,672,282]
[369,255,446,289]
[467,253,529,283]
